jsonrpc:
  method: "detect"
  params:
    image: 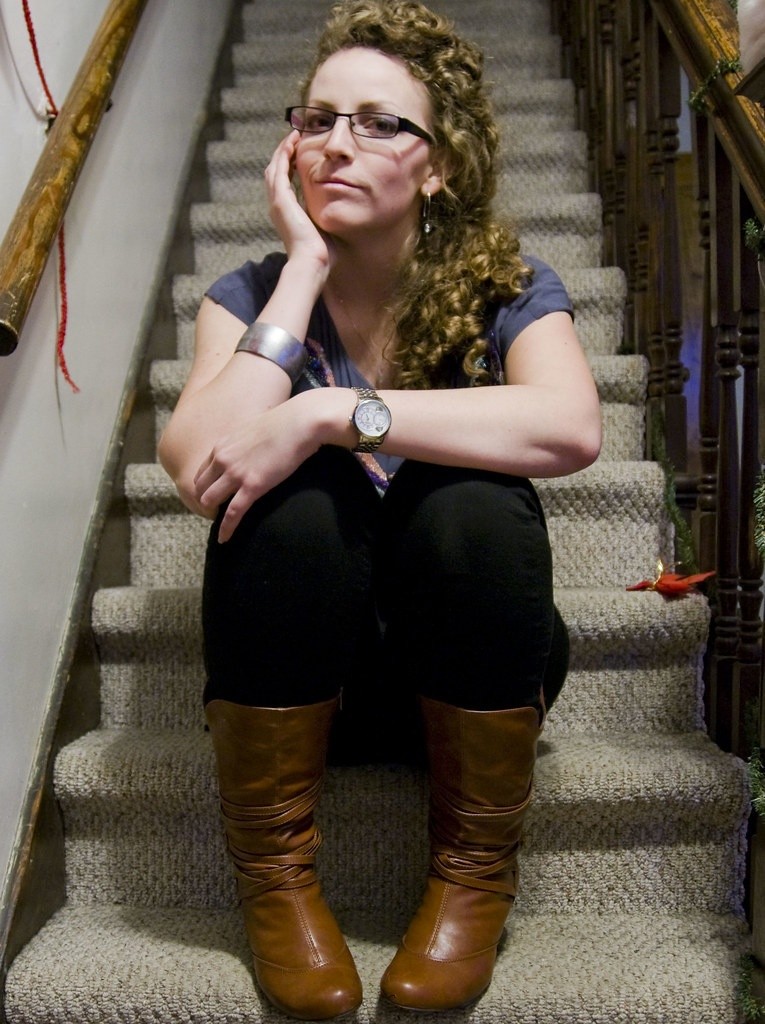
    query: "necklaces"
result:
[329,275,392,389]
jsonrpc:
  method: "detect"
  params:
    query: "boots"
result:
[381,680,547,1013]
[200,668,363,1021]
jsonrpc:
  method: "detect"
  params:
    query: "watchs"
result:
[351,387,391,452]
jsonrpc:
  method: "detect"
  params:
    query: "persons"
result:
[160,0,603,1024]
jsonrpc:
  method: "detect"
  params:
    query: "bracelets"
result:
[234,322,308,386]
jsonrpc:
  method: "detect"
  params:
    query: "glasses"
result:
[284,104,435,146]
